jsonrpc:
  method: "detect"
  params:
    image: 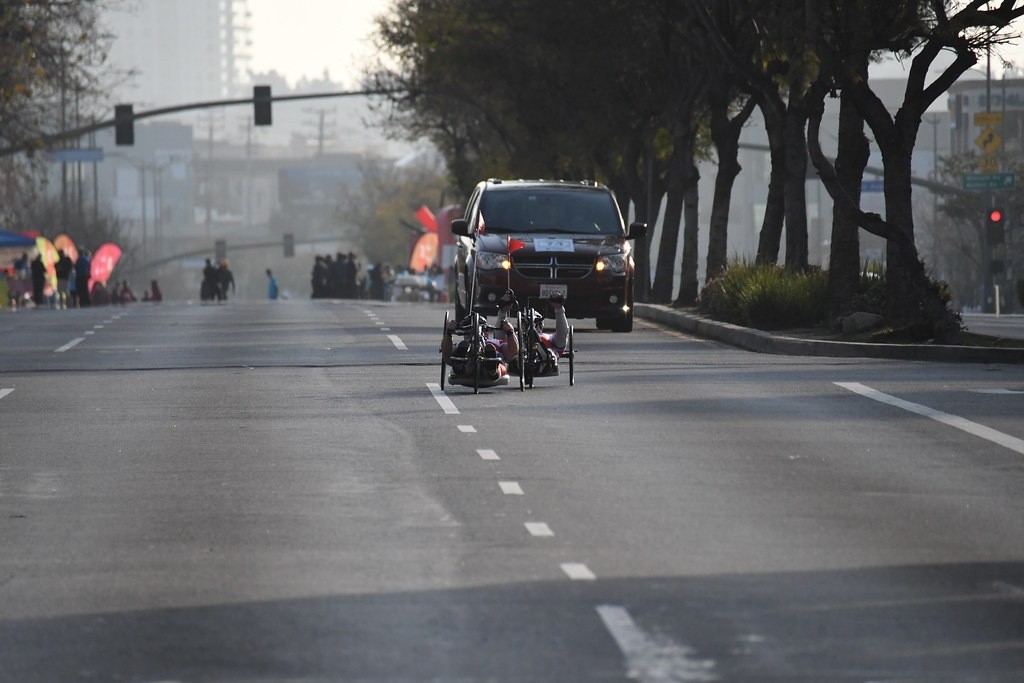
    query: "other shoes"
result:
[453,340,469,367]
[485,343,497,378]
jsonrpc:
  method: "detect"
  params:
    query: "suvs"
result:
[450,178,649,333]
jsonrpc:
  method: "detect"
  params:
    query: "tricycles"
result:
[438,293,578,394]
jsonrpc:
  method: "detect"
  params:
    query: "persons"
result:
[265,269,278,300]
[308,251,449,304]
[0,250,163,310]
[442,290,572,381]
[200,257,236,302]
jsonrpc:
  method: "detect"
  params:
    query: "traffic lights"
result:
[987,206,1005,246]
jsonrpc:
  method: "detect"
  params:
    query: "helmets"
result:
[521,311,544,328]
[459,315,488,331]
